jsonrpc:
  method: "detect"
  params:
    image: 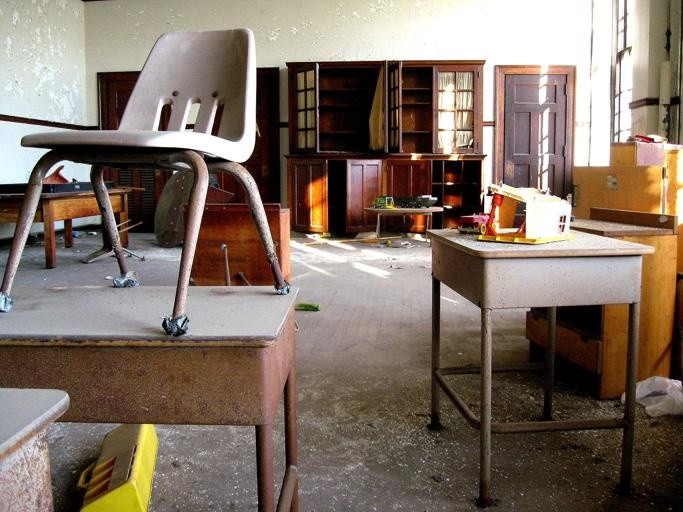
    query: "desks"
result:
[1,186,133,269]
[425,227,655,508]
[1,388,71,511]
[1,283,303,511]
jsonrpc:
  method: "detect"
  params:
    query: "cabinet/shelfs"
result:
[286,60,390,154]
[96,67,280,232]
[293,156,384,235]
[390,59,486,155]
[388,157,482,236]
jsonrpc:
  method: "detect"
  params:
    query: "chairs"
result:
[0,27,290,336]
